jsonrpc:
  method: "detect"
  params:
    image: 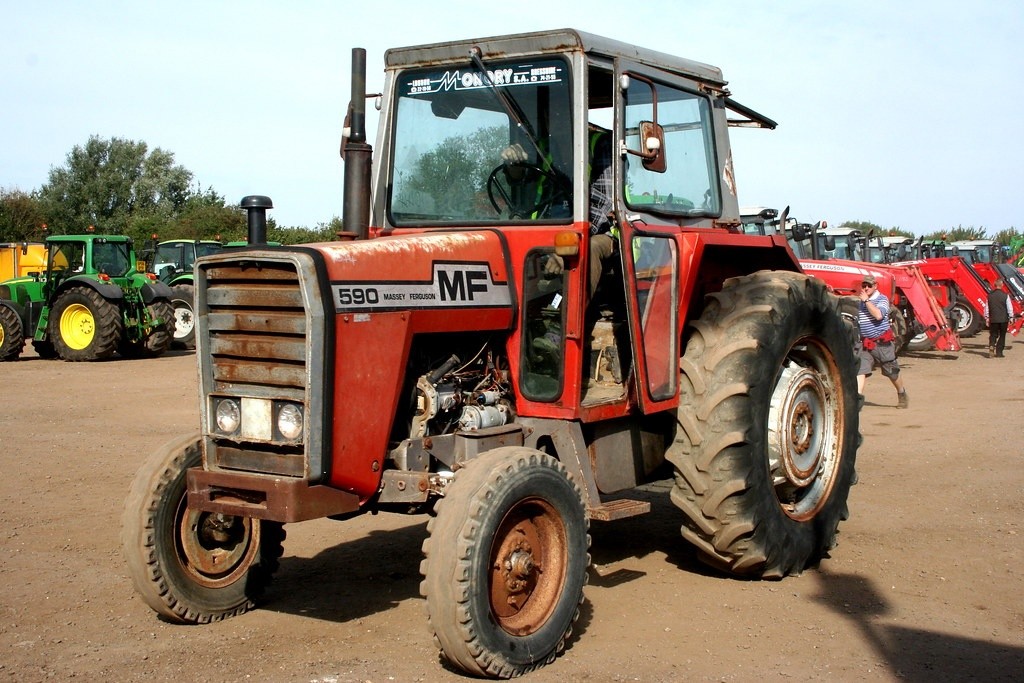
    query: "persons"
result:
[984,278,1014,358]
[853,274,909,409]
[501,93,630,376]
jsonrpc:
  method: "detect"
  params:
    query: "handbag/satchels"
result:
[861,328,894,351]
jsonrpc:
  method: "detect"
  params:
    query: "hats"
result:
[862,276,876,284]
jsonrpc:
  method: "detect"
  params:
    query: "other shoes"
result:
[988,346,995,358]
[996,355,1005,358]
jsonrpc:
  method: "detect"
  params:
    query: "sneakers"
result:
[532,333,561,351]
[897,388,909,408]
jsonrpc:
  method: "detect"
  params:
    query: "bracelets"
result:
[1008,317,1013,318]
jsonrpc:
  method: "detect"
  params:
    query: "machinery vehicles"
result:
[124,27,863,680]
[584,193,1024,358]
[0,234,176,362]
[147,239,282,348]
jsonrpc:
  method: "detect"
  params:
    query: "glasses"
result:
[862,285,873,288]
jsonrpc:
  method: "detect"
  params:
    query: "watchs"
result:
[864,299,870,303]
